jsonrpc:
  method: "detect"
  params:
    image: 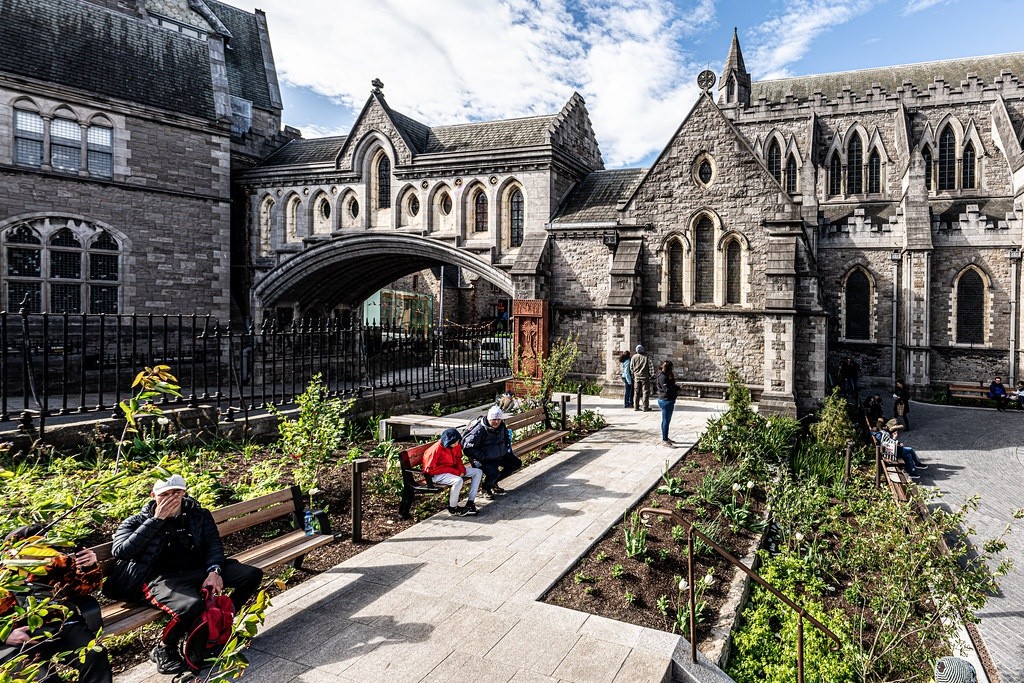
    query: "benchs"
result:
[881,460,954,578]
[1,486,339,682]
[864,409,906,489]
[947,383,1018,404]
[399,399,570,519]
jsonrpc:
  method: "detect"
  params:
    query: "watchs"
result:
[209,566,222,574]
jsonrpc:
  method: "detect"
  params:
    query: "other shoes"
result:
[997,408,1007,413]
[487,477,504,493]
[481,482,495,500]
[635,409,642,411]
[647,408,652,411]
[464,503,476,514]
[903,428,910,432]
[908,471,920,479]
[662,438,676,448]
[914,462,928,469]
[446,505,470,517]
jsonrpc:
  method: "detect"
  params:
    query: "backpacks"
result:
[460,415,488,449]
[178,588,235,670]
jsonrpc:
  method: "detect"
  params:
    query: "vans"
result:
[479,337,505,367]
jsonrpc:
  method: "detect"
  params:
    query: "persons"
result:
[893,378,910,432]
[656,360,685,448]
[990,377,1024,413]
[619,345,655,412]
[0,522,110,683]
[463,405,522,500]
[421,427,483,517]
[102,473,264,674]
[862,392,928,478]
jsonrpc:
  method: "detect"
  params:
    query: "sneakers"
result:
[150,641,183,675]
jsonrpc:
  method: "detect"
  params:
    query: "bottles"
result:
[304,511,314,536]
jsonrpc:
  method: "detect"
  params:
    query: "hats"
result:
[153,474,186,496]
[635,345,645,353]
[4,524,47,546]
[487,405,503,421]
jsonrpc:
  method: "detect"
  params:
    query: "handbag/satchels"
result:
[897,400,904,416]
